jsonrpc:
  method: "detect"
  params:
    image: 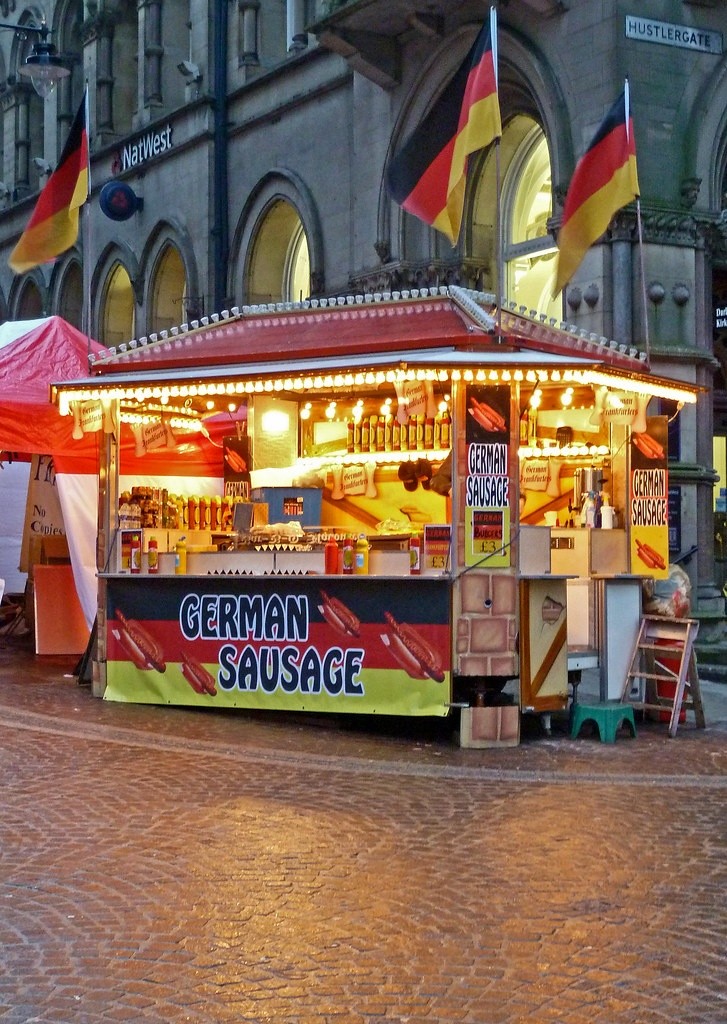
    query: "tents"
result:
[0,316,247,635]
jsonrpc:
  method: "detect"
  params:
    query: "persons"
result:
[429,448,453,498]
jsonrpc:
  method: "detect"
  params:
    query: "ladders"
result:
[620,613,707,737]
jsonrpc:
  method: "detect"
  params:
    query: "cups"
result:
[545,511,557,527]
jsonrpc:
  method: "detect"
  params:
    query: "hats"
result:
[398,461,418,491]
[414,458,432,490]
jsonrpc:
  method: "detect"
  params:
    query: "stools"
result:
[570,702,637,745]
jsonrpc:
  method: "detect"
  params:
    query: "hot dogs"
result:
[633,433,665,460]
[318,589,361,638]
[225,446,247,472]
[385,611,445,683]
[469,397,507,432]
[111,609,166,673]
[179,650,217,697]
[635,538,666,570]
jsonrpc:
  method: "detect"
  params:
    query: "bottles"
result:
[410,533,421,575]
[600,496,613,529]
[148,536,158,574]
[119,500,132,529]
[175,536,187,575]
[342,534,354,574]
[353,534,368,574]
[346,412,449,451]
[585,491,596,527]
[130,536,141,574]
[324,536,338,574]
[130,500,141,529]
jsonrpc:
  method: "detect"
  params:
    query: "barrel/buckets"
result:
[569,466,608,517]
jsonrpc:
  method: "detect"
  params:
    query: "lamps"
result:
[0,181,8,199]
[33,158,52,176]
[177,60,199,84]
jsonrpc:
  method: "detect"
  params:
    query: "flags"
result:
[387,10,502,246]
[7,89,88,273]
[552,91,639,299]
